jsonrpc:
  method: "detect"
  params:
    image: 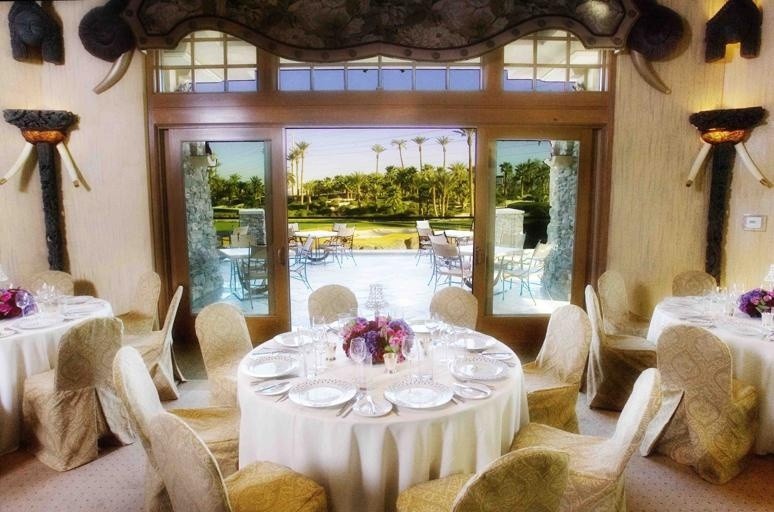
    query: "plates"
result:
[351,399,395,419]
[253,380,292,397]
[454,331,497,350]
[453,383,492,401]
[289,378,356,409]
[382,378,454,410]
[450,356,510,381]
[246,356,298,380]
[275,330,318,347]
[1,295,94,338]
[664,295,761,337]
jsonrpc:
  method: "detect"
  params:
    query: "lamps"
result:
[0,106,87,271]
[678,103,769,284]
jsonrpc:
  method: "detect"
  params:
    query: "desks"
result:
[1,287,113,350]
[655,295,773,363]
[238,316,518,425]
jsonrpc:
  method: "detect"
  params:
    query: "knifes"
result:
[367,395,376,414]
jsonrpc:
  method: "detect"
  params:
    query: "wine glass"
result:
[15,290,30,319]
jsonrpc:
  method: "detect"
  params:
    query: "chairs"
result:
[597,268,718,338]
[220,210,554,331]
[23,316,129,449]
[17,272,162,337]
[121,284,184,397]
[649,324,759,482]
[520,304,592,435]
[147,414,326,511]
[585,283,654,409]
[112,344,242,510]
[195,303,255,406]
[397,447,567,511]
[509,367,663,512]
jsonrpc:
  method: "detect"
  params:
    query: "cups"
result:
[358,352,374,390]
[700,282,745,299]
[761,312,774,340]
[301,306,444,378]
